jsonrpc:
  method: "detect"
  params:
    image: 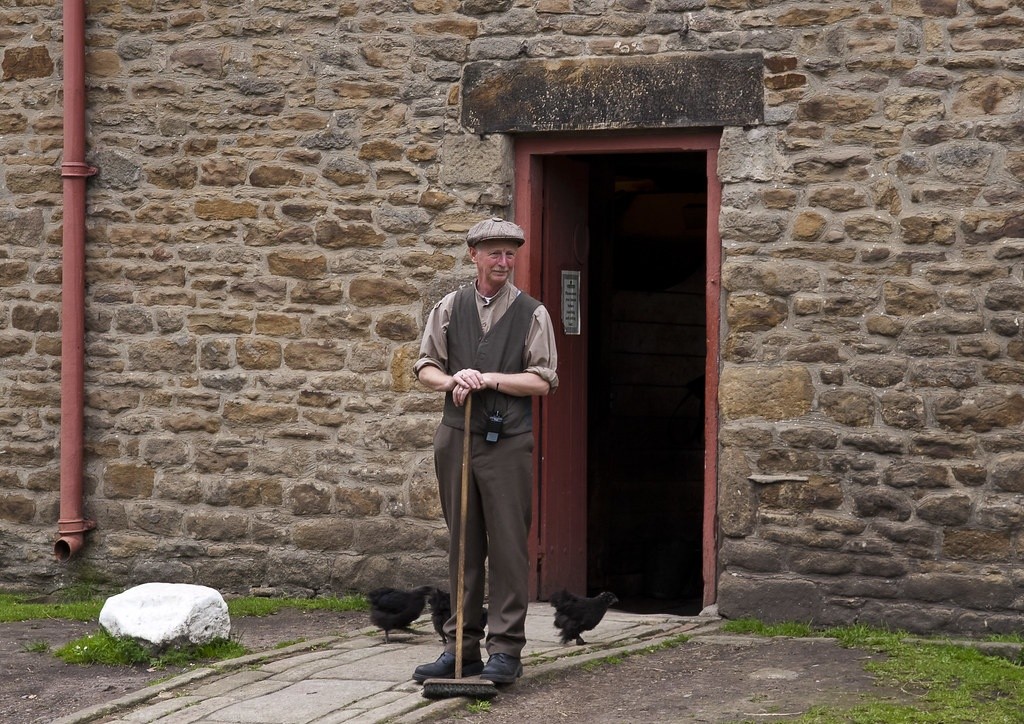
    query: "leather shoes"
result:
[480,653,523,683]
[412,651,484,682]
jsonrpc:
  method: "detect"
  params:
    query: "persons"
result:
[413,215,561,682]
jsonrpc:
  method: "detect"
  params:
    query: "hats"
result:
[466,218,525,248]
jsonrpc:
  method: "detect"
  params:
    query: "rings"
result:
[459,388,463,391]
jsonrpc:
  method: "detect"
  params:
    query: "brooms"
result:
[422,389,501,702]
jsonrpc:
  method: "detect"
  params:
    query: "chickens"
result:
[428,590,488,645]
[549,590,619,646]
[365,586,433,644]
